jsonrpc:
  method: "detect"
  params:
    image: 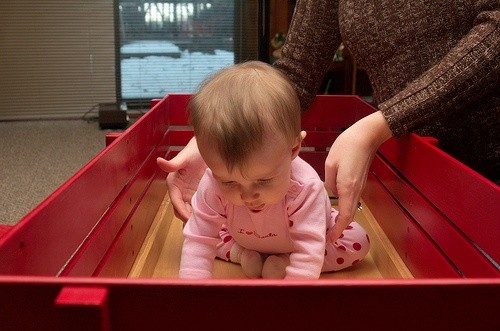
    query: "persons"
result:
[157,0,500,241]
[171,61,370,281]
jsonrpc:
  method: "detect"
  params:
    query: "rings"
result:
[355,201,362,212]
[184,201,191,204]
